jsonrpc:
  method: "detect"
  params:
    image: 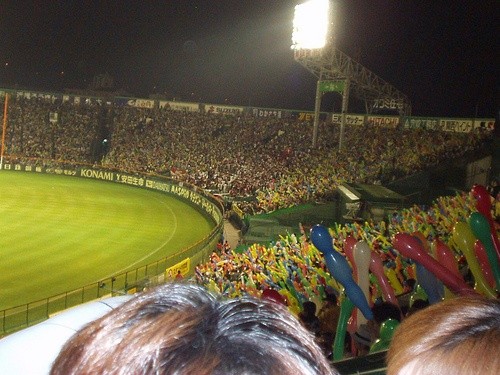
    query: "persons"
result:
[46,278,341,375]
[0,89,499,361]
[384,298,500,374]
